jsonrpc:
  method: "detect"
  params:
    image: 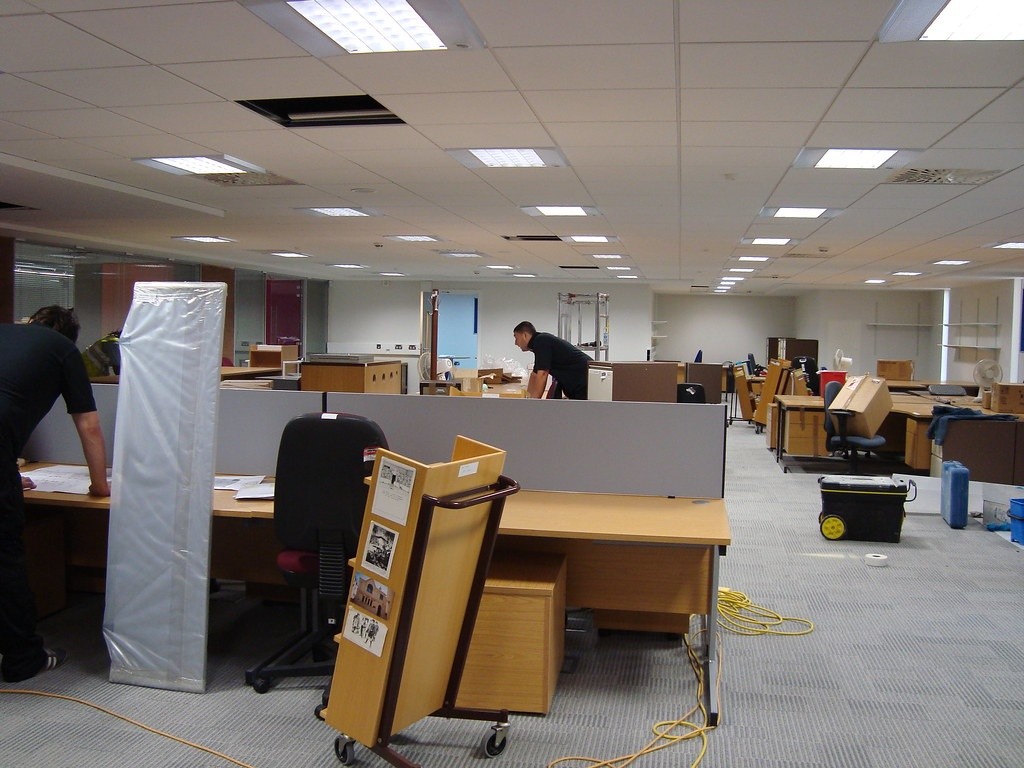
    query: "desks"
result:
[746,375,1024,421]
[301,360,402,395]
[254,376,301,391]
[221,366,282,381]
[17,463,733,723]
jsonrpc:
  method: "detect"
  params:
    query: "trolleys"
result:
[726,365,753,426]
[334,476,523,768]
[754,366,793,433]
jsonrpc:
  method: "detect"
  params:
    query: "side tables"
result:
[991,382,1024,414]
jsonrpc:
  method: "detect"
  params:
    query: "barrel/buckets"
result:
[940,460,970,528]
[1006,498,1024,546]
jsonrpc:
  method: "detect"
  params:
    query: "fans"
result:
[417,352,453,381]
[973,358,1003,400]
[833,348,854,379]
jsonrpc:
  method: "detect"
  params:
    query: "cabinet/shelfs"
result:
[904,418,932,471]
[937,323,1001,350]
[767,337,796,365]
[249,344,299,371]
[929,420,1016,485]
[587,360,678,404]
[683,362,723,404]
[557,291,610,362]
[766,403,778,448]
[651,321,668,338]
[786,338,818,364]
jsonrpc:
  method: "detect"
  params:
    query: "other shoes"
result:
[38,648,67,671]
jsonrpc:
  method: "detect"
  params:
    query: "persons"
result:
[0,304,112,683]
[351,613,379,647]
[81,330,121,384]
[513,320,594,400]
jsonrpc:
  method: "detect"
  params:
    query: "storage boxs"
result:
[828,375,894,440]
[1007,498,1024,545]
[817,476,917,544]
[982,391,991,409]
[453,551,571,715]
[820,371,848,398]
[877,358,913,381]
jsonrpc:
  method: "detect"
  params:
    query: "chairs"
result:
[823,381,886,476]
[244,410,390,719]
[677,383,706,404]
